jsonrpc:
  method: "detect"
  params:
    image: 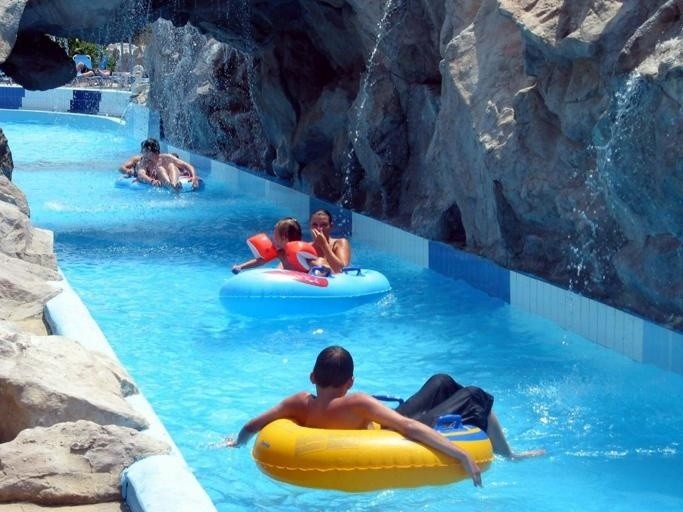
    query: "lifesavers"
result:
[114,175,204,195]
[247,232,318,274]
[220,267,392,319]
[252,396,494,491]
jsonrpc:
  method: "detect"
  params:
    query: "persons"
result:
[75,62,111,77]
[118,152,180,178]
[135,138,199,193]
[230,217,321,277]
[276,208,350,274]
[224,345,548,489]
